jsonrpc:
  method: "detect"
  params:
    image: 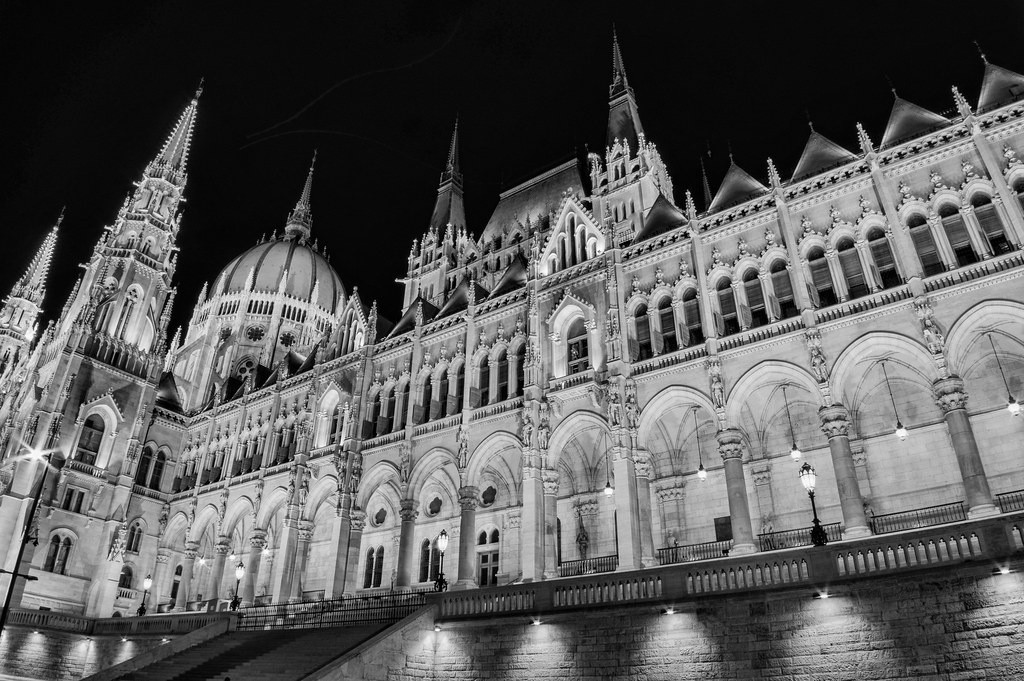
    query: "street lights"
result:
[136,574,153,615]
[433,528,449,593]
[228,562,247,611]
[797,461,829,547]
